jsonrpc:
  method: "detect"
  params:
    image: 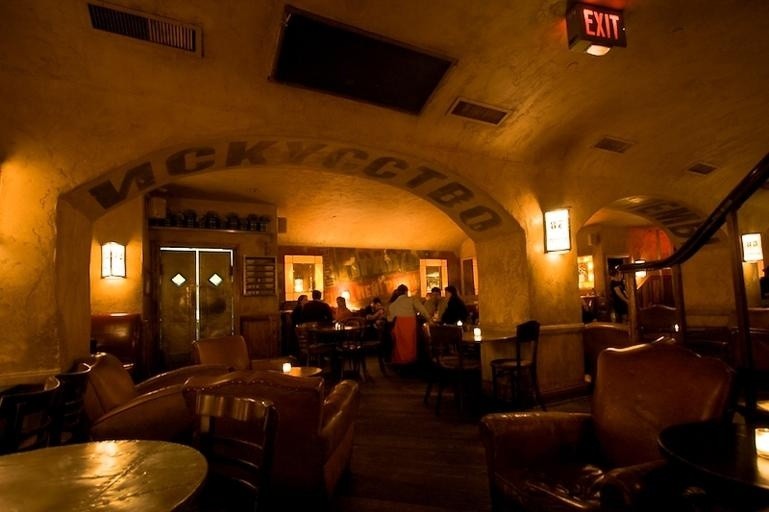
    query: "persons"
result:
[760,267,766,299]
[608,269,633,324]
[280,284,476,385]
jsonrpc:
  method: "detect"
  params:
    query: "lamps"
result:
[101,241,127,279]
[543,207,572,253]
[741,232,764,263]
